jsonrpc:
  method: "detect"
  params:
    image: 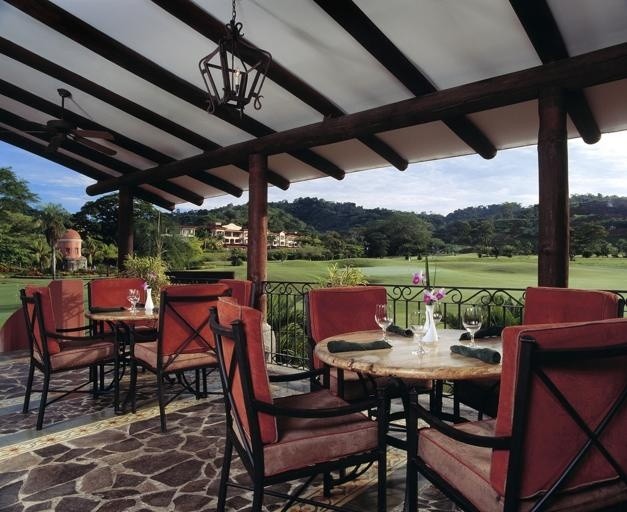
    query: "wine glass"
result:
[410,310,430,354]
[433,303,442,327]
[375,305,394,345]
[127,288,141,314]
[462,307,482,348]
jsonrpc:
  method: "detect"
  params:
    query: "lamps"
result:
[198,0,272,119]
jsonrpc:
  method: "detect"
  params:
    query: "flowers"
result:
[142,271,175,290]
[412,254,444,306]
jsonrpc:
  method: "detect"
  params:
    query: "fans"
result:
[24,89,117,156]
